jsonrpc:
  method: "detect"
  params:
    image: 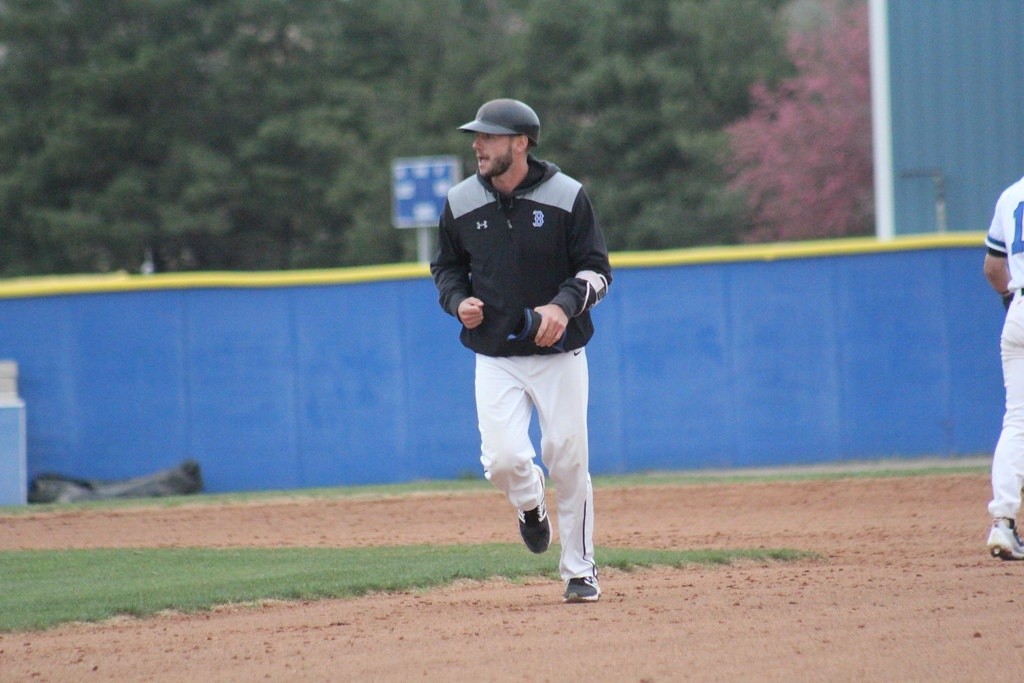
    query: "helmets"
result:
[456,98,540,147]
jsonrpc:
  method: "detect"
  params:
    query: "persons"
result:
[431,99,614,601]
[983,170,1024,561]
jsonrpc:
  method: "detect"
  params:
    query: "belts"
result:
[1021,288,1024,296]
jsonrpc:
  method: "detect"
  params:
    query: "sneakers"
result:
[986,517,1024,560]
[561,575,601,603]
[517,464,553,554]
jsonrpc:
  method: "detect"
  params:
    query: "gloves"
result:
[1002,290,1014,312]
